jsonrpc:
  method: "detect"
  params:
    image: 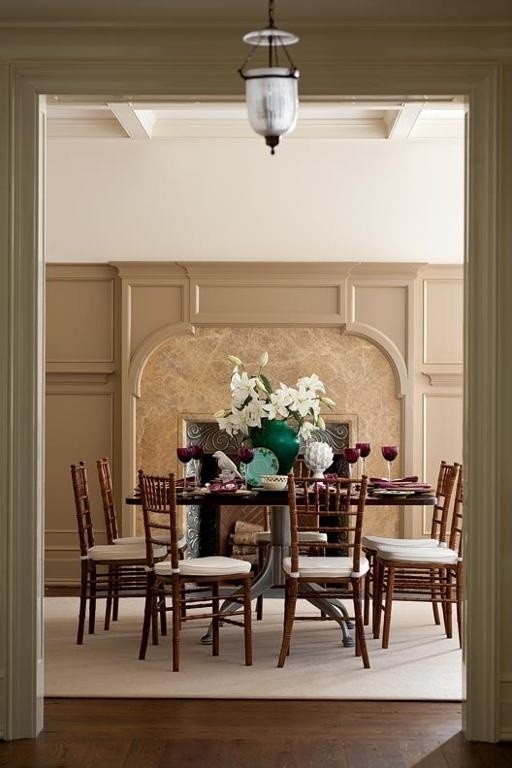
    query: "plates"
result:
[253,486,291,492]
[373,490,416,496]
[160,486,184,490]
[239,446,280,486]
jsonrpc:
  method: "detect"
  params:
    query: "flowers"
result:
[213,348,334,441]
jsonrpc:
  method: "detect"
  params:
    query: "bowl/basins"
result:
[259,475,288,490]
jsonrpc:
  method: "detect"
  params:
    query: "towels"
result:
[156,478,191,488]
[207,480,239,493]
[310,473,432,492]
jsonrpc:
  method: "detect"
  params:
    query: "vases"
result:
[252,414,303,474]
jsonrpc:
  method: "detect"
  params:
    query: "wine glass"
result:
[189,445,202,490]
[237,447,254,492]
[382,446,397,483]
[356,443,370,477]
[343,448,360,496]
[176,447,193,497]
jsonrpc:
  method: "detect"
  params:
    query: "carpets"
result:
[42,596,464,700]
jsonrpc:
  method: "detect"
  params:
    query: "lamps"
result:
[236,1,301,155]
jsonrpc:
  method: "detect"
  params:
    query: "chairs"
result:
[70,460,169,646]
[135,470,254,672]
[372,464,464,650]
[362,460,461,634]
[252,457,330,622]
[96,456,189,632]
[278,473,369,670]
